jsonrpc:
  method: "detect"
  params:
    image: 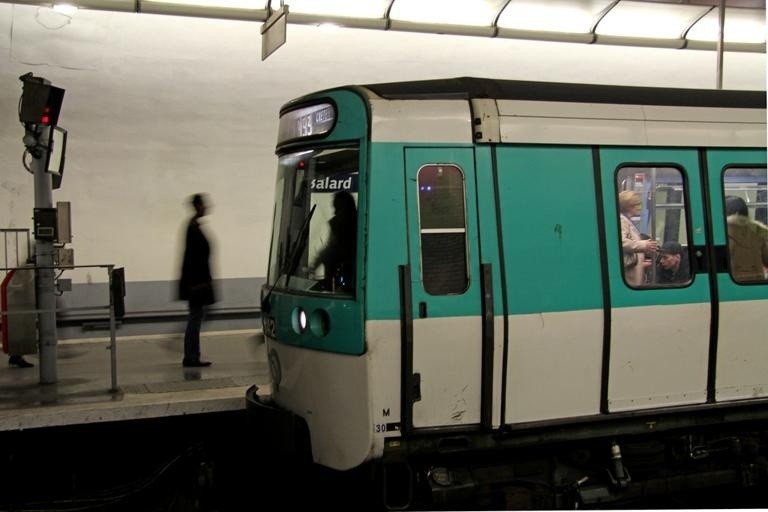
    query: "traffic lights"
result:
[17,71,66,127]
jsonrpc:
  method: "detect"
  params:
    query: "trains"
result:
[246,72,768,510]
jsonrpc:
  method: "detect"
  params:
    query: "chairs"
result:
[656,246,693,283]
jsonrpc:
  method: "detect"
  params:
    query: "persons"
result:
[0,242,70,370]
[176,191,222,367]
[726,196,768,282]
[657,240,691,284]
[619,191,659,288]
[306,189,358,293]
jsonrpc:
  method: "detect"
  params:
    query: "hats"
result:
[655,239,683,256]
